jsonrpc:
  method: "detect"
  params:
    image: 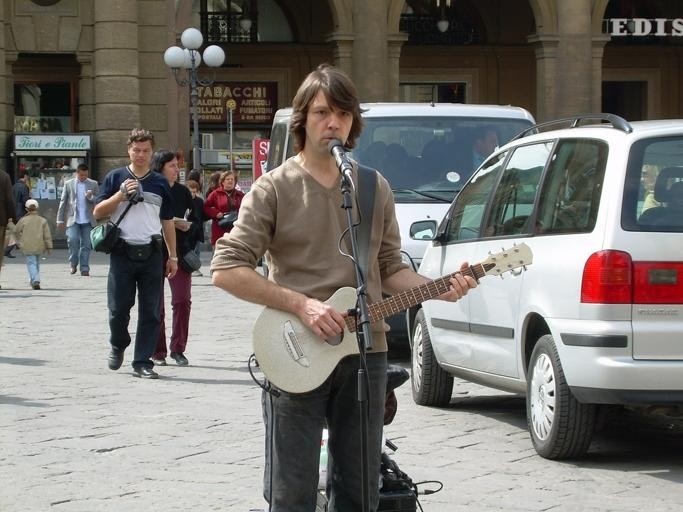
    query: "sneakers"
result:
[32,283,40,290]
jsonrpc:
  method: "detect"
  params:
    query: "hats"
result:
[25,199,39,208]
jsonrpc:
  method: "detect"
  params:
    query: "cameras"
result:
[126,181,145,204]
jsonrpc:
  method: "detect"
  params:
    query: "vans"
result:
[265,101,543,352]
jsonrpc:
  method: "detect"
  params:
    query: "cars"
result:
[405,113,682,461]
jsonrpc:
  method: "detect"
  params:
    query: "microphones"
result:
[327,139,354,177]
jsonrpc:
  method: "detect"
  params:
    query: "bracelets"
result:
[167,254,179,261]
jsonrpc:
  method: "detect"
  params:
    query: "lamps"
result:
[434,1,453,32]
[238,1,253,33]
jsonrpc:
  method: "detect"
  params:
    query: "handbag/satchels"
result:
[181,250,202,274]
[89,221,121,255]
[125,243,153,262]
[218,210,238,229]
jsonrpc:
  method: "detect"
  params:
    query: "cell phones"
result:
[87,190,92,193]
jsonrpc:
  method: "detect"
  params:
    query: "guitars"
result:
[254,243,533,393]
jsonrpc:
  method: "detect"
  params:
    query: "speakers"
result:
[315,454,416,512]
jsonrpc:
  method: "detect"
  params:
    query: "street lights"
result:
[163,28,225,171]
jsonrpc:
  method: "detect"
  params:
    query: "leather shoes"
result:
[81,271,89,276]
[108,345,126,370]
[132,366,158,379]
[170,352,188,365]
[152,358,166,366]
[70,265,77,274]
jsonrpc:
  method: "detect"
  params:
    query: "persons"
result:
[0,164,246,292]
[206,59,483,510]
[92,125,179,380]
[465,127,501,173]
[145,146,195,365]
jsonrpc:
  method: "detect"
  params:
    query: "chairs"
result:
[638,166,683,226]
